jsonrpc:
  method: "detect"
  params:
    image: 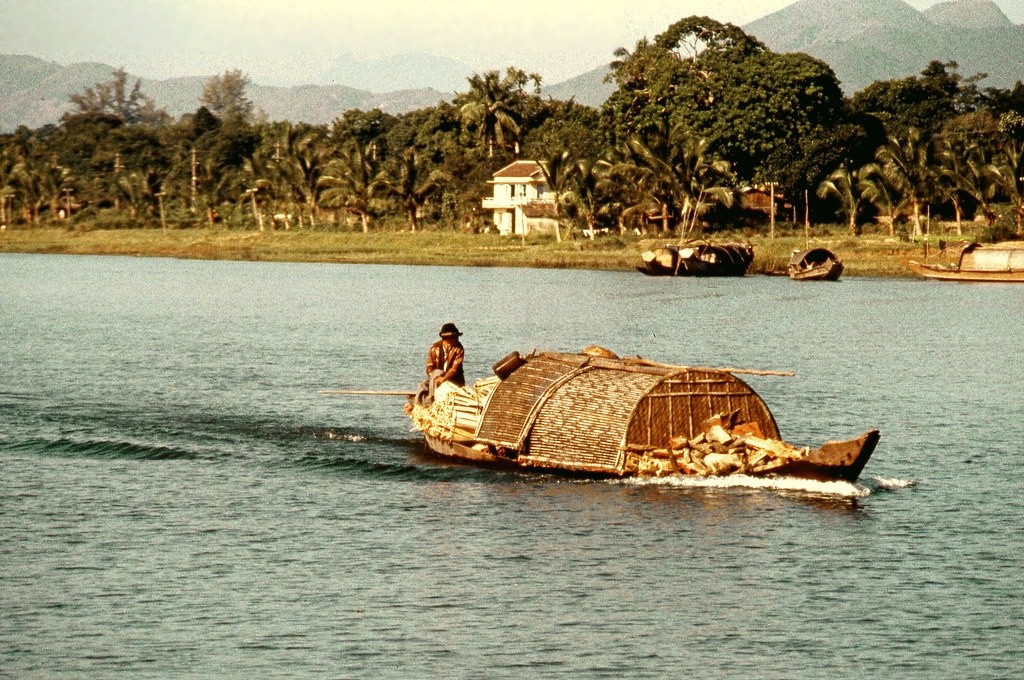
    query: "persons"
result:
[425,323,464,386]
[937,238,947,258]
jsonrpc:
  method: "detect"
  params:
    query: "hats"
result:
[439,323,463,338]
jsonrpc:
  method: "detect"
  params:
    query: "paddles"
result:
[581,343,795,377]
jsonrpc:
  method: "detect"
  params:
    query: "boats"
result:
[406,346,881,494]
[635,240,757,278]
[788,248,845,280]
[906,243,1024,284]
